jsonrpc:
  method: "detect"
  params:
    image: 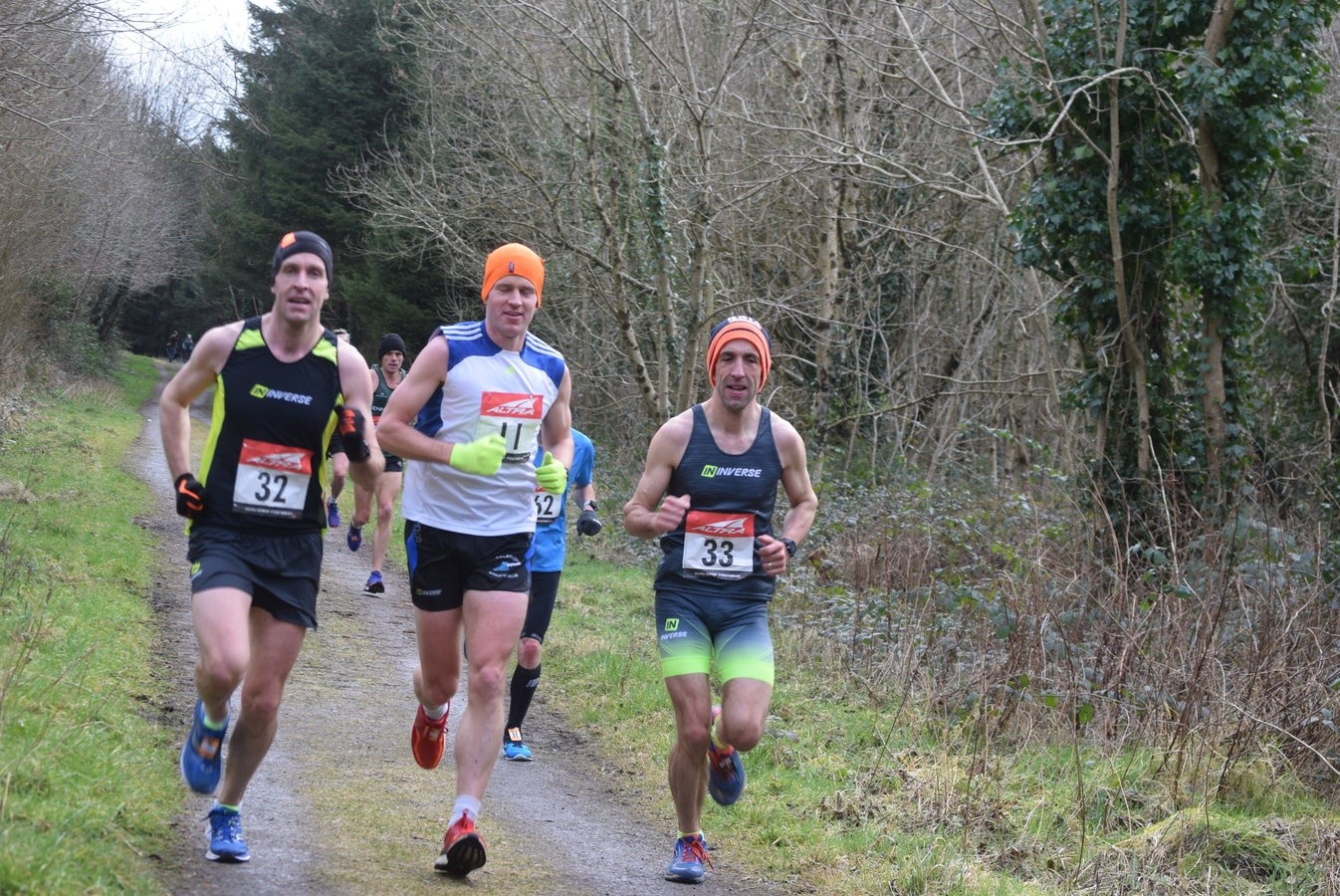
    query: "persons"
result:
[623,314,819,885]
[376,242,572,874]
[326,327,350,527]
[347,332,419,597]
[464,427,603,760]
[159,232,385,865]
[166,329,192,362]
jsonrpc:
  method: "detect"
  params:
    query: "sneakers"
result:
[348,521,363,552]
[411,698,451,769]
[327,497,340,528]
[433,807,488,876]
[364,571,384,593]
[502,740,533,761]
[707,705,745,806]
[205,807,250,864]
[181,696,230,796]
[664,832,714,884]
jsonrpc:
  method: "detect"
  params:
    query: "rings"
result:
[768,562,772,570]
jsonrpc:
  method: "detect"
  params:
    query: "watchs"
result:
[776,538,797,559]
[582,501,599,511]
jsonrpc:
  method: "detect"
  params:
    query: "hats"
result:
[270,231,333,290]
[482,243,544,309]
[379,334,406,361]
[707,315,772,391]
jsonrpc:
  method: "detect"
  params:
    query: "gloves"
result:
[334,403,370,462]
[535,451,567,495]
[449,432,507,477]
[174,473,207,519]
[577,510,603,537]
